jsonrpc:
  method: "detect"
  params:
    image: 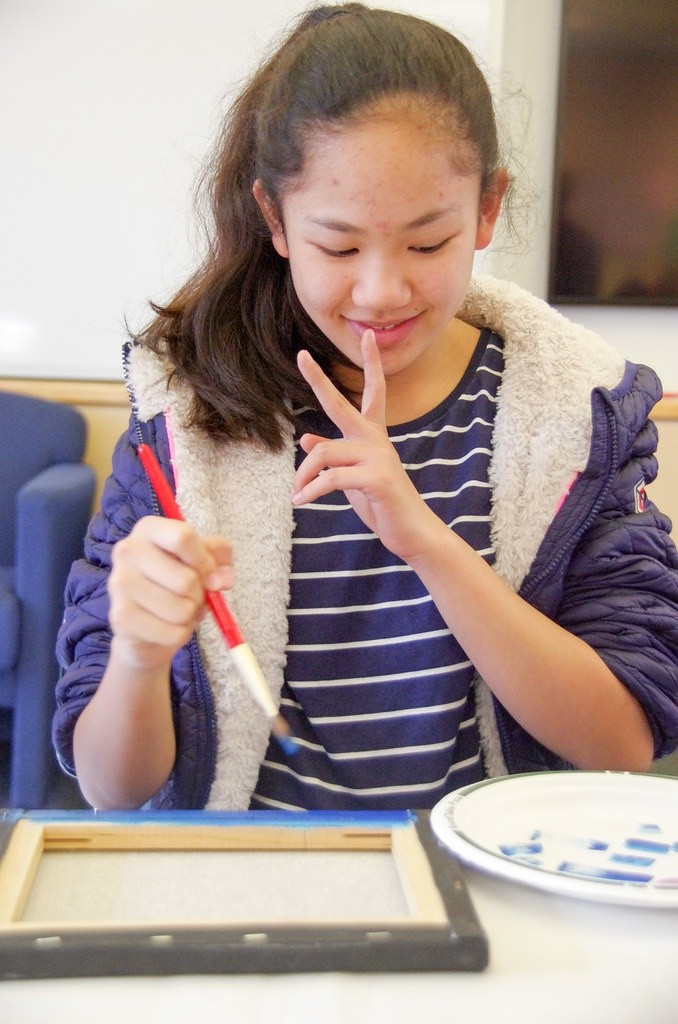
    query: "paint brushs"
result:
[137,443,301,755]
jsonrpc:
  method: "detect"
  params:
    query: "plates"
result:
[430,769,678,908]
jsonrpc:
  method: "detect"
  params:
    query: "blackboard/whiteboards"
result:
[1,1,507,408]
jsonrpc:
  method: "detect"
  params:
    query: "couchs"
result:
[0,390,100,810]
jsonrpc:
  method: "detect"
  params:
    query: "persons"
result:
[52,0,678,811]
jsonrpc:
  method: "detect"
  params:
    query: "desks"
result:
[0,808,678,1024]
